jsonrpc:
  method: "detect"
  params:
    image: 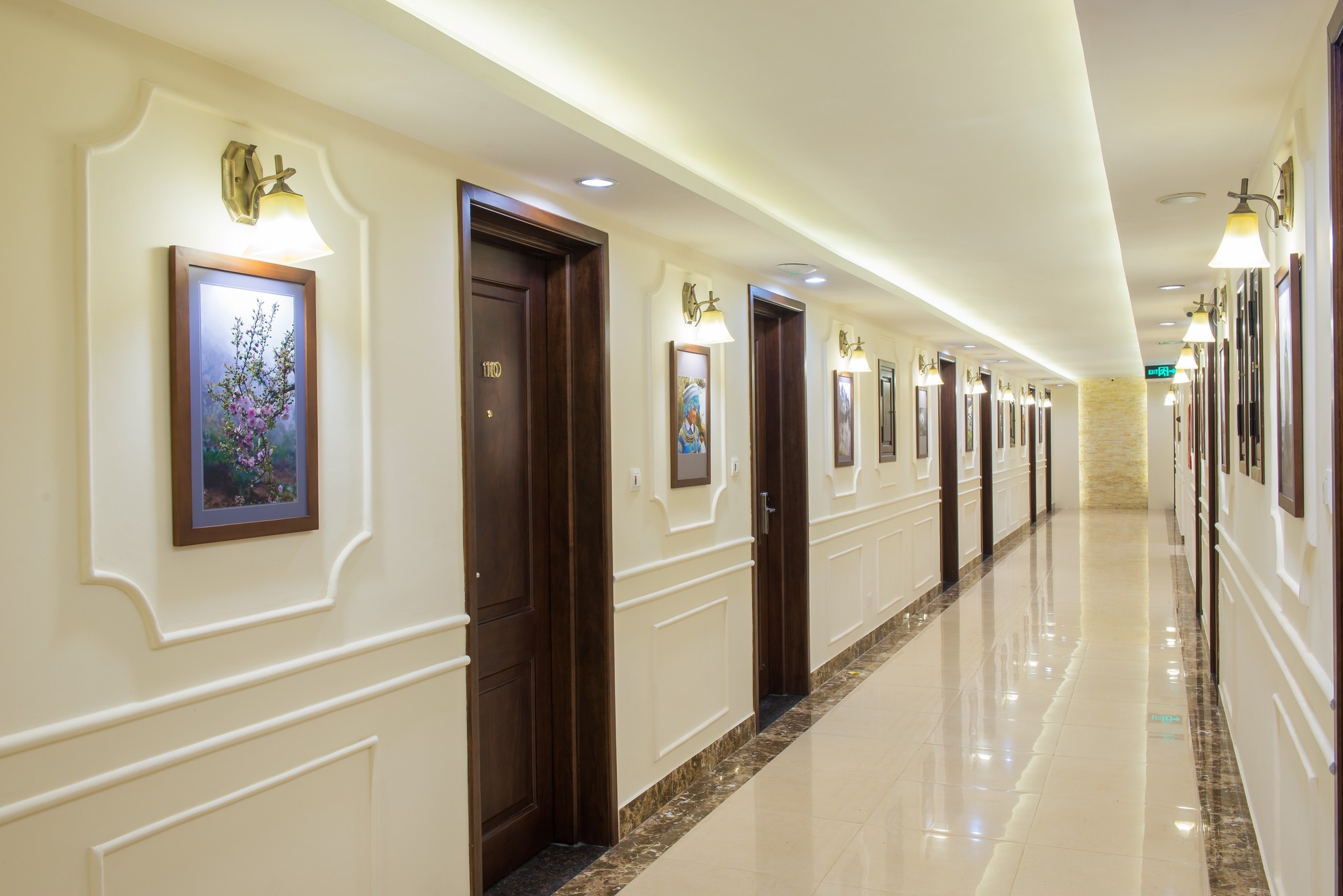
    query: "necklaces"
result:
[683,422,696,442]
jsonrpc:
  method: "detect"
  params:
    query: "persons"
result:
[678,381,701,454]
[699,431,706,453]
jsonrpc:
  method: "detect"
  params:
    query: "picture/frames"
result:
[834,369,854,468]
[671,341,711,489]
[168,246,320,546]
[916,385,929,458]
[965,394,973,452]
[1192,253,1300,520]
[997,400,1043,448]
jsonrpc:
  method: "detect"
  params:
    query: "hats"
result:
[682,383,700,419]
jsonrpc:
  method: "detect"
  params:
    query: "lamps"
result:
[1182,284,1227,342]
[1164,384,1178,405]
[999,379,1015,400]
[1175,342,1204,369]
[1040,392,1052,407]
[839,330,872,372]
[682,283,735,345]
[919,354,944,385]
[1022,387,1035,405]
[1172,368,1190,383]
[1208,156,1293,268]
[967,369,987,394]
[221,140,334,264]
